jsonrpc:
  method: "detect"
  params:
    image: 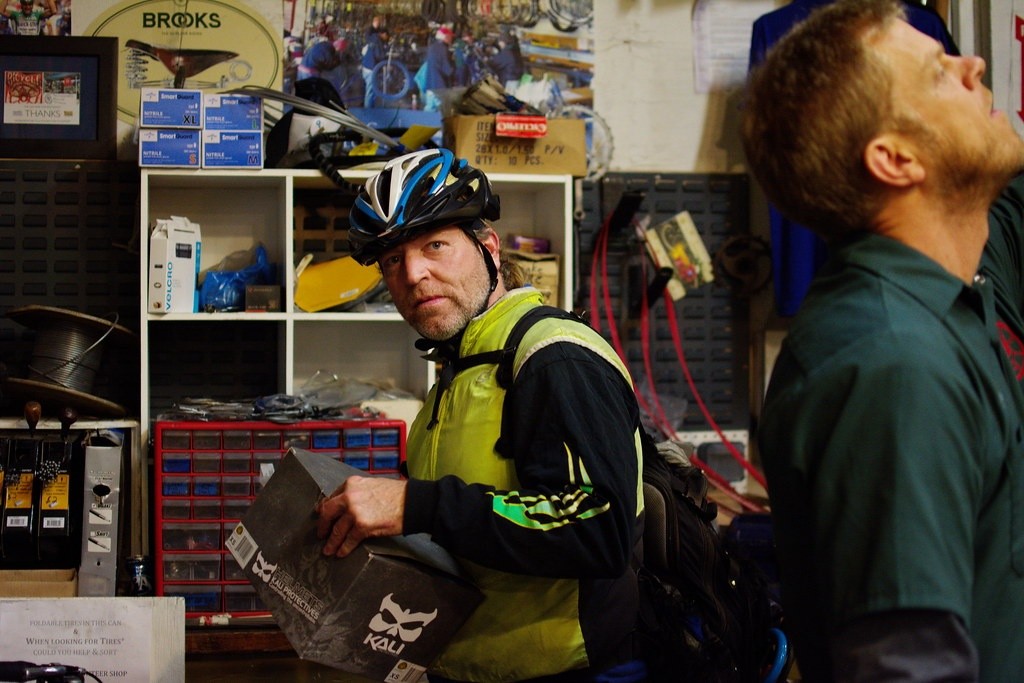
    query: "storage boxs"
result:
[502,247,560,308]
[139,87,265,169]
[446,113,587,178]
[148,216,201,313]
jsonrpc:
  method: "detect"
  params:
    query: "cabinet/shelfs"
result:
[137,167,575,683]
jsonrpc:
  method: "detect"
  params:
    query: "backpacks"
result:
[499,306,722,683]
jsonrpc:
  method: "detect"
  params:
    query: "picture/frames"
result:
[0,36,118,161]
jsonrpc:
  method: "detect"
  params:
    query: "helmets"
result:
[348,148,500,266]
[20,0,33,4]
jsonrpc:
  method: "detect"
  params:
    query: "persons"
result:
[739,0,1024,683]
[0,0,71,36]
[283,14,520,111]
[314,147,652,682]
